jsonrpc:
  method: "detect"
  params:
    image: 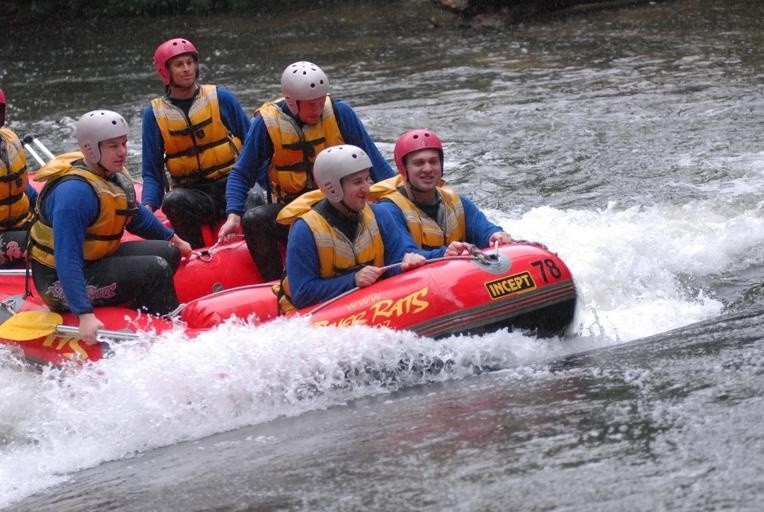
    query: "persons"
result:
[141,38,272,249]
[27,111,192,345]
[272,145,426,316]
[218,61,397,281]
[376,128,512,259]
[0,88,40,268]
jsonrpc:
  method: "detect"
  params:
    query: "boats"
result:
[1,169,579,375]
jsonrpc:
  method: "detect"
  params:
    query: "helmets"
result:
[394,129,443,178]
[281,61,330,116]
[314,144,373,203]
[153,38,199,85]
[76,110,129,164]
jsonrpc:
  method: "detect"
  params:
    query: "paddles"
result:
[0,311,140,341]
